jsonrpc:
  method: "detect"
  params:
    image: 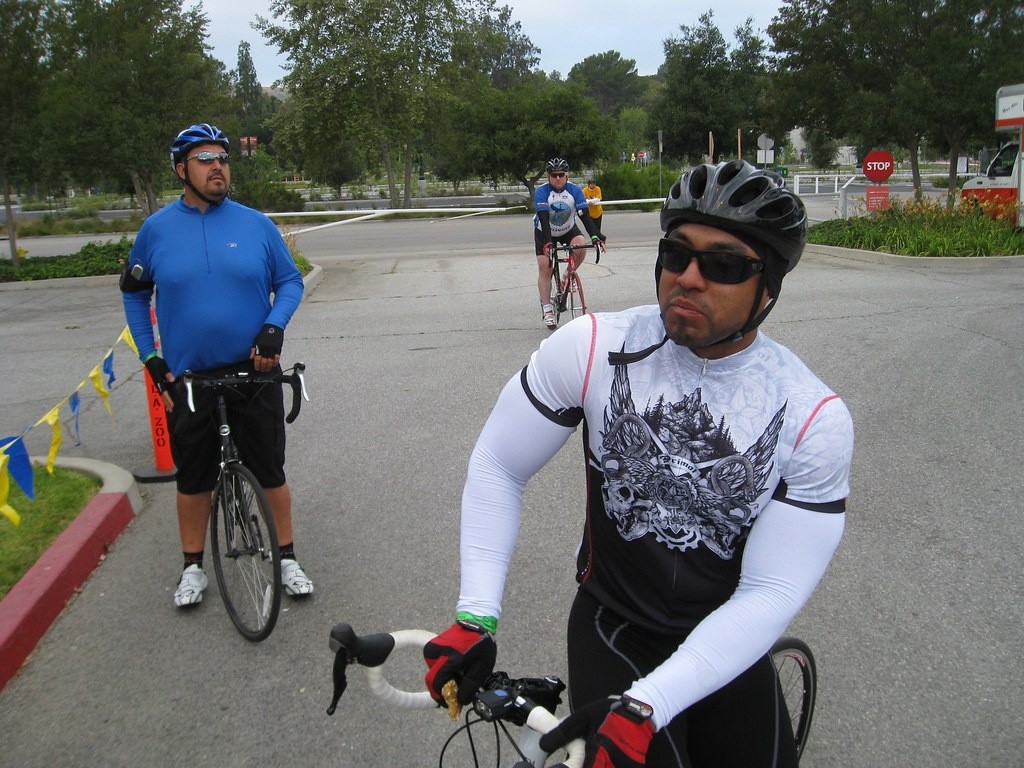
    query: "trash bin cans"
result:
[782,168,788,178]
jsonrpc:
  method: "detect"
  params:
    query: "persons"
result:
[424,161,854,768]
[582,178,603,233]
[120,123,314,606]
[532,157,607,325]
[623,146,651,168]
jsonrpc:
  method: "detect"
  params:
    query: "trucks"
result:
[960,83,1024,229]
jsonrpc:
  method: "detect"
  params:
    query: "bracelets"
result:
[142,350,157,364]
[592,236,599,240]
[456,611,497,635]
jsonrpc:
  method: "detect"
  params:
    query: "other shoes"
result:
[563,270,578,293]
[543,304,555,325]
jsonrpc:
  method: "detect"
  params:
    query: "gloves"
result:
[539,695,655,768]
[423,620,497,709]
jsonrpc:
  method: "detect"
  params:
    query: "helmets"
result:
[546,158,569,173]
[660,159,808,297]
[169,123,229,181]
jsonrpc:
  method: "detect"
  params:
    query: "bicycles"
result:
[182,361,310,644]
[326,621,821,768]
[541,238,602,331]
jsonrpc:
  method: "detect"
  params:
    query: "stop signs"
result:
[638,151,645,158]
[863,147,894,183]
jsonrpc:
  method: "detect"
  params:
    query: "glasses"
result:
[549,173,565,178]
[658,237,764,284]
[181,151,231,166]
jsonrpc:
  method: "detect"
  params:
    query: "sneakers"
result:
[174,563,208,607]
[280,559,313,596]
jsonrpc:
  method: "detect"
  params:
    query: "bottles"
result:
[560,267,569,291]
